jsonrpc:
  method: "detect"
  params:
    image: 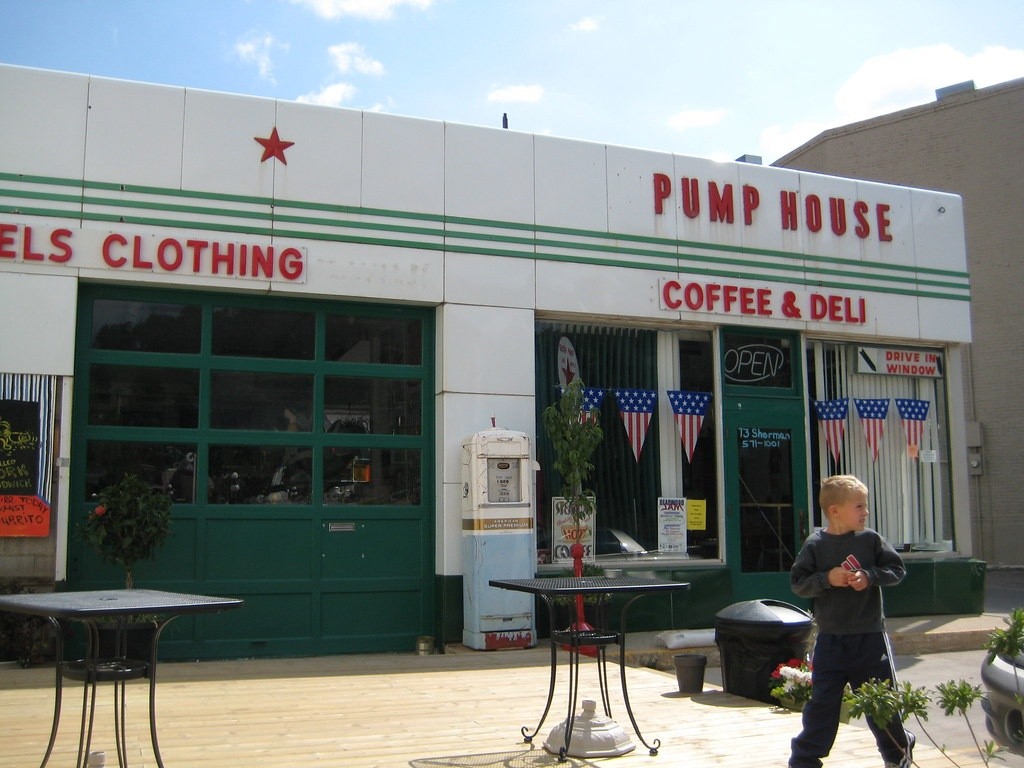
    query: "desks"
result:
[488,576,691,763]
[0,590,243,768]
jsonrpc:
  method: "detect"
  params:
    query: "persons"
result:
[790,474,917,768]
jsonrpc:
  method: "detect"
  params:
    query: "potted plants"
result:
[549,560,617,630]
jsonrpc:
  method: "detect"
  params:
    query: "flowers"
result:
[767,657,853,708]
[54,472,180,638]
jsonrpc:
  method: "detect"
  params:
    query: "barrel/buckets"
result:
[672,653,707,693]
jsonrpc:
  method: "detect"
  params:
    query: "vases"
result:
[779,697,855,724]
[56,617,163,668]
[673,654,707,694]
